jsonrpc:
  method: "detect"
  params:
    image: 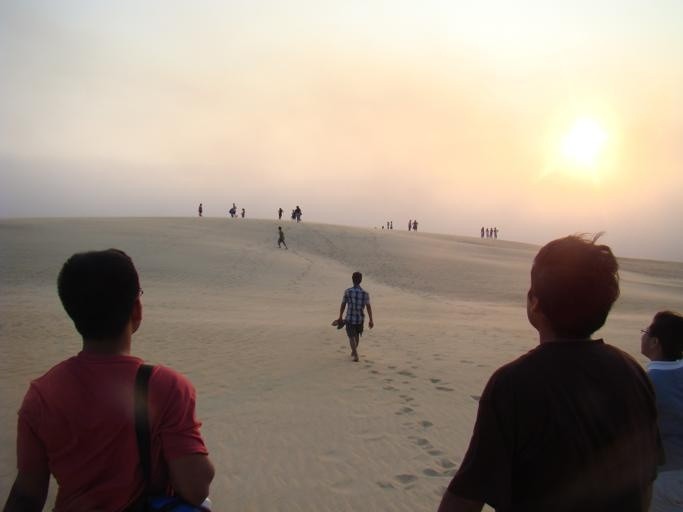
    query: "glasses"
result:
[137,288,143,296]
[639,329,650,336]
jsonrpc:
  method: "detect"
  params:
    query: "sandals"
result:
[331,320,339,326]
[337,319,345,329]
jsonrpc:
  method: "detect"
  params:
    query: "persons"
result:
[278,206,302,222]
[278,227,287,248]
[338,271,374,363]
[481,227,497,239]
[408,219,418,231]
[230,203,245,218]
[638,310,683,511]
[0,244,216,511]
[435,229,666,512]
[199,203,202,216]
[387,221,392,229]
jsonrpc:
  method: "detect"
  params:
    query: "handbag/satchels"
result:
[133,363,212,512]
